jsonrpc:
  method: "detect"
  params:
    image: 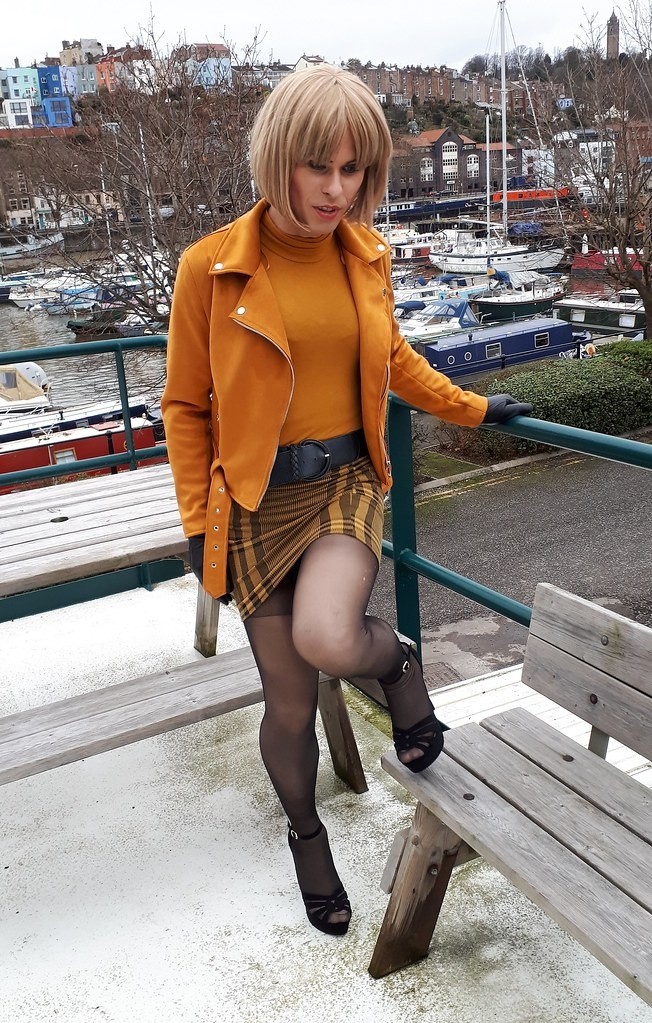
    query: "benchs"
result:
[0,461,367,792]
[365,582,652,1006]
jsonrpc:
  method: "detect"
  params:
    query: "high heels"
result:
[285,822,352,937]
[378,642,445,775]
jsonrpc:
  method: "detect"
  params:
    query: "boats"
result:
[2,188,652,495]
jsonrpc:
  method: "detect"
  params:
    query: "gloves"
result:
[188,533,234,606]
[482,393,534,426]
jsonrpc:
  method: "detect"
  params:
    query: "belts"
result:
[265,427,368,488]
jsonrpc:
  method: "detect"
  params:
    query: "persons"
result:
[162,64,535,934]
